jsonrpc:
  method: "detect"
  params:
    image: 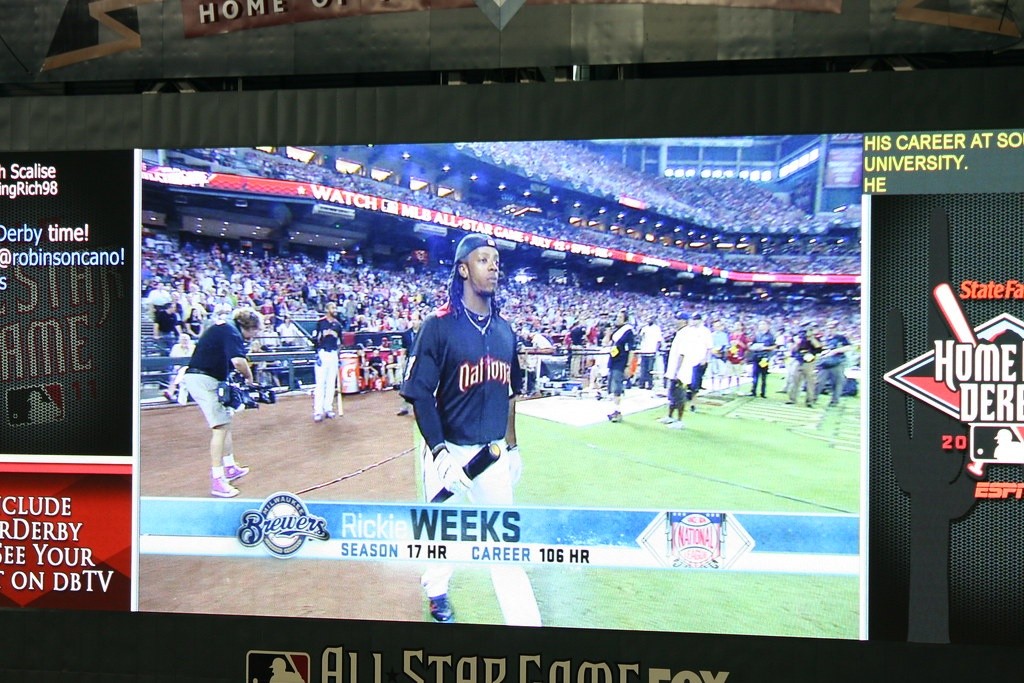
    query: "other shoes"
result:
[760,393,767,397]
[807,403,814,408]
[656,414,674,423]
[668,421,682,429]
[785,401,795,404]
[429,597,453,623]
[749,393,756,395]
[315,413,323,422]
[327,411,336,417]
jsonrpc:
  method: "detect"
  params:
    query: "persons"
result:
[140,141,861,429]
[183,306,261,497]
[398,233,543,627]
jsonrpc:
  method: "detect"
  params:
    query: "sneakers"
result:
[224,463,250,480]
[211,478,238,497]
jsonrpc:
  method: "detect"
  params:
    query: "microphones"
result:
[263,384,274,389]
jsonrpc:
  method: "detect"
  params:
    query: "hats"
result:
[690,312,702,320]
[455,233,496,263]
[672,311,688,320]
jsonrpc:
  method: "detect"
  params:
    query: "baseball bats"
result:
[337,371,344,418]
[428,442,502,504]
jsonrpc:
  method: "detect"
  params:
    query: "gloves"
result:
[435,450,472,496]
[509,445,521,486]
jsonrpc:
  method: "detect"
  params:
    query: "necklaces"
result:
[461,299,492,335]
